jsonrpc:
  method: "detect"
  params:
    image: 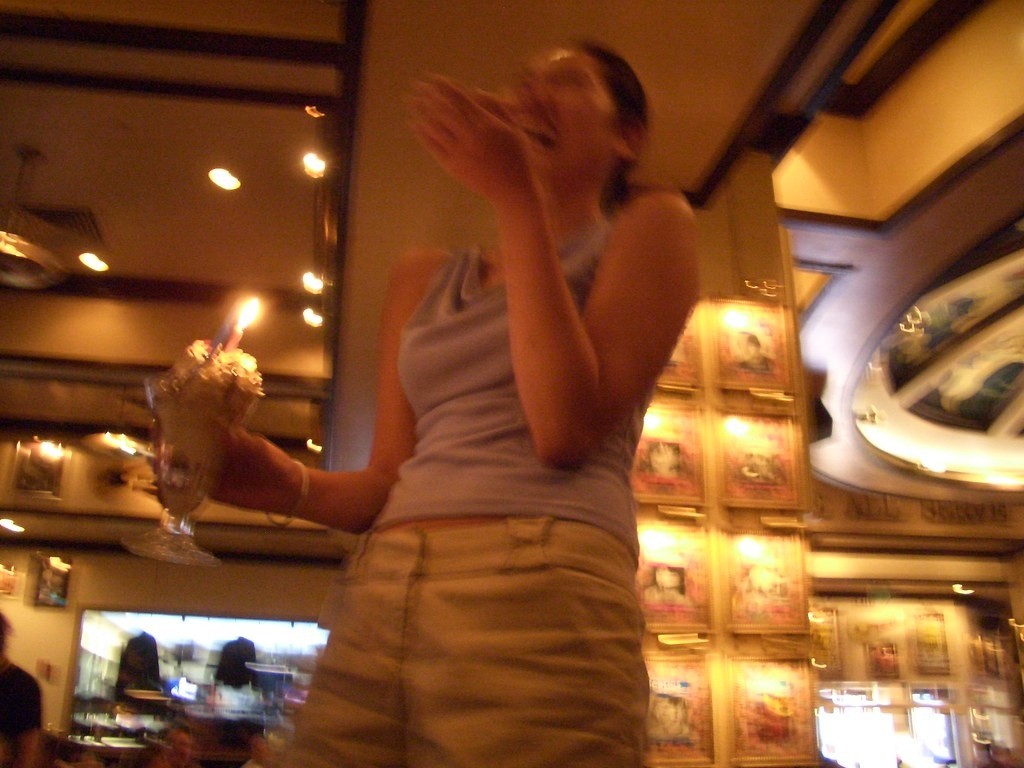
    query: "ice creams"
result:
[145,337,266,518]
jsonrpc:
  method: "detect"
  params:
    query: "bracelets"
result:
[266,458,309,526]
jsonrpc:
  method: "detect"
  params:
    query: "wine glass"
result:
[120,377,262,570]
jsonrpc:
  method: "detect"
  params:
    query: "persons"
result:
[206,40,701,767]
[0,613,43,768]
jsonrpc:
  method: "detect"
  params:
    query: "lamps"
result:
[0,143,75,292]
